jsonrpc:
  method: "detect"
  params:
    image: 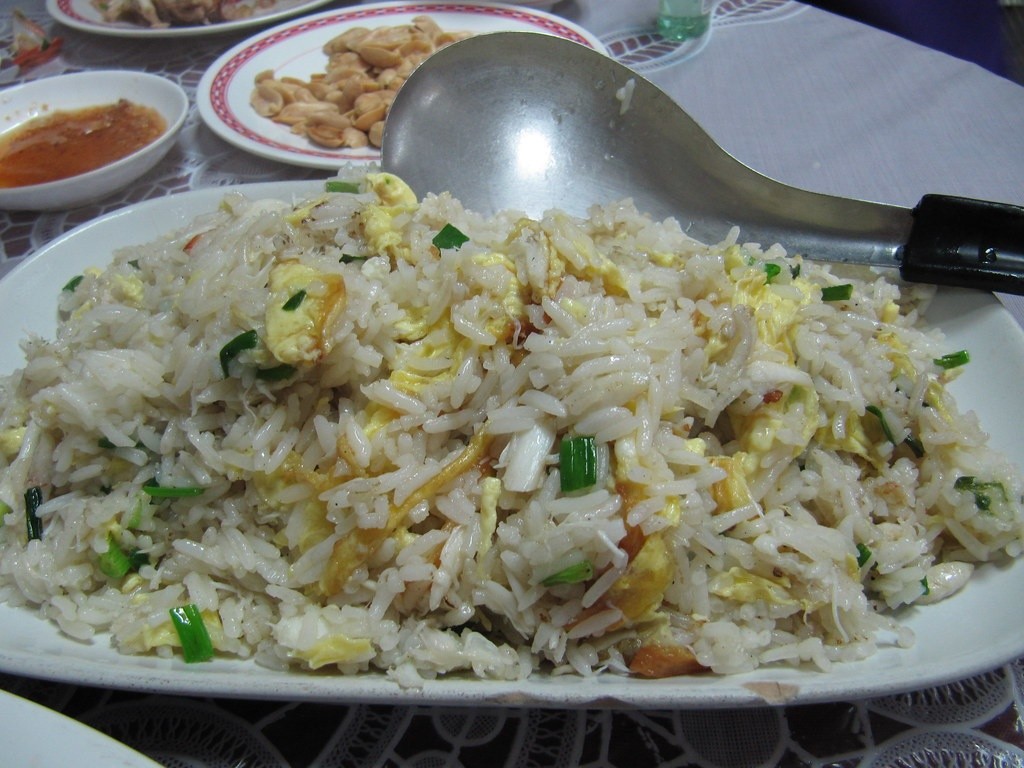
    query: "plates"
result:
[44,0,337,40]
[196,0,608,174]
[0,176,1023,705]
[1,689,167,768]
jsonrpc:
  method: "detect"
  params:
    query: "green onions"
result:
[26,182,1009,663]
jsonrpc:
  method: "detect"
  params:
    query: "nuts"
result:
[250,16,476,149]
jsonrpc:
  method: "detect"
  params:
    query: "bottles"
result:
[655,0,714,40]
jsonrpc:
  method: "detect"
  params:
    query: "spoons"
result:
[380,31,1024,303]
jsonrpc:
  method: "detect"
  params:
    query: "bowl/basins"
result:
[0,68,192,216]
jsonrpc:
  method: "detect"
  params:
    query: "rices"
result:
[0,160,1024,687]
